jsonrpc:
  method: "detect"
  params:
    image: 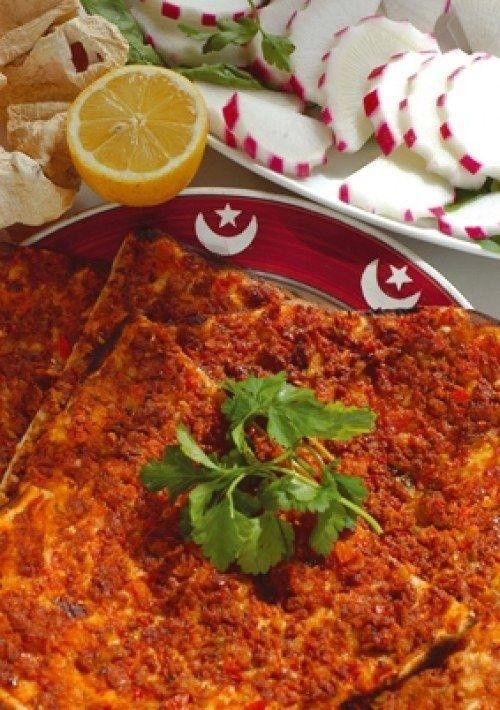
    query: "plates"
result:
[204,132,500,261]
[14,185,475,328]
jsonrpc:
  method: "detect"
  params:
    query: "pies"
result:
[0,234,500,710]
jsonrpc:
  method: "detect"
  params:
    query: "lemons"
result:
[66,64,209,208]
